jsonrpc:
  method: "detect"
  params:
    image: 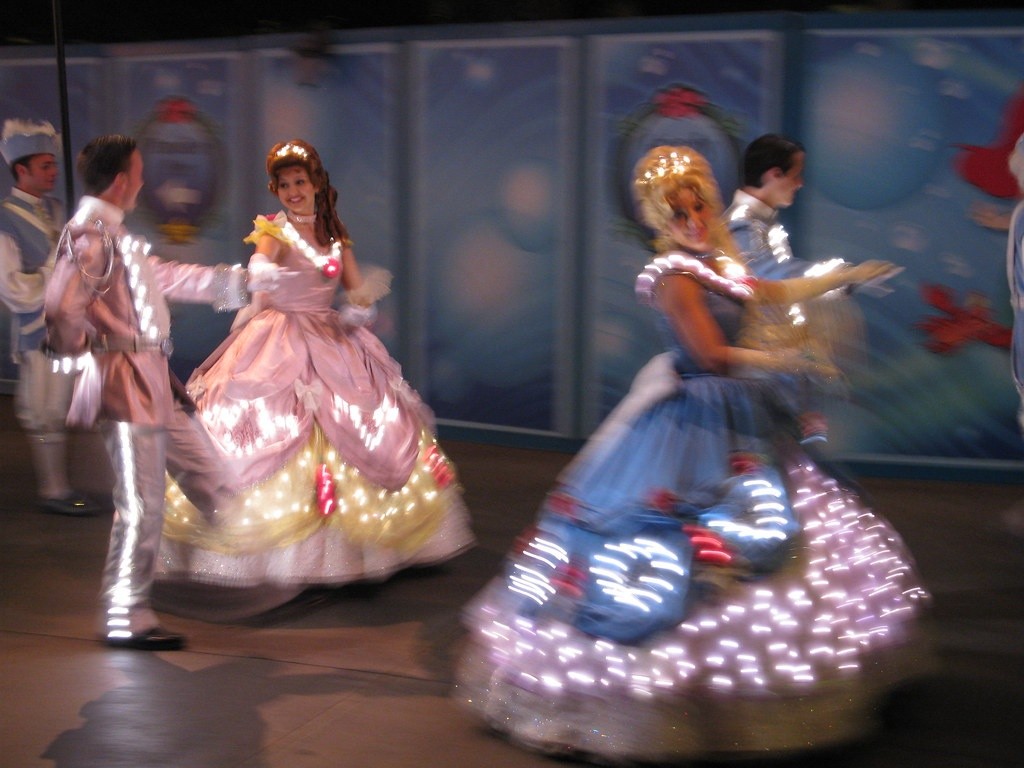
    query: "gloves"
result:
[779,258,907,302]
[249,253,292,282]
[341,303,380,327]
[736,347,841,379]
[248,271,300,293]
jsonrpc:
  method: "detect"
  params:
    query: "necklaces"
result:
[288,210,318,223]
[694,253,713,259]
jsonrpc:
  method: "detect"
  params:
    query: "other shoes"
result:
[105,624,188,650]
[34,487,115,516]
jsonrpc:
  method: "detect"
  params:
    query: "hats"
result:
[0,119,59,164]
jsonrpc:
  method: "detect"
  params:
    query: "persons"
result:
[44,134,300,650]
[713,133,906,338]
[152,139,479,594]
[448,144,932,762]
[0,119,107,517]
